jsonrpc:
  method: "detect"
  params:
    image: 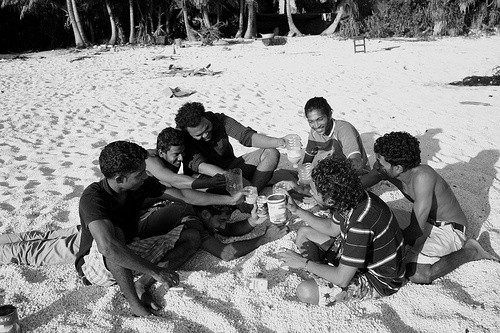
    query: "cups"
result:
[268,195,287,223]
[273,182,289,195]
[244,186,258,205]
[287,137,300,151]
[224,169,243,196]
[297,162,311,180]
[255,194,266,217]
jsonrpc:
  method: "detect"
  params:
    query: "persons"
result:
[279,155,408,304]
[291,130,498,284]
[293,97,373,199]
[1,187,294,267]
[178,101,306,199]
[136,126,228,215]
[76,138,254,323]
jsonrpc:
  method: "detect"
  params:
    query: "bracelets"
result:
[305,259,310,274]
